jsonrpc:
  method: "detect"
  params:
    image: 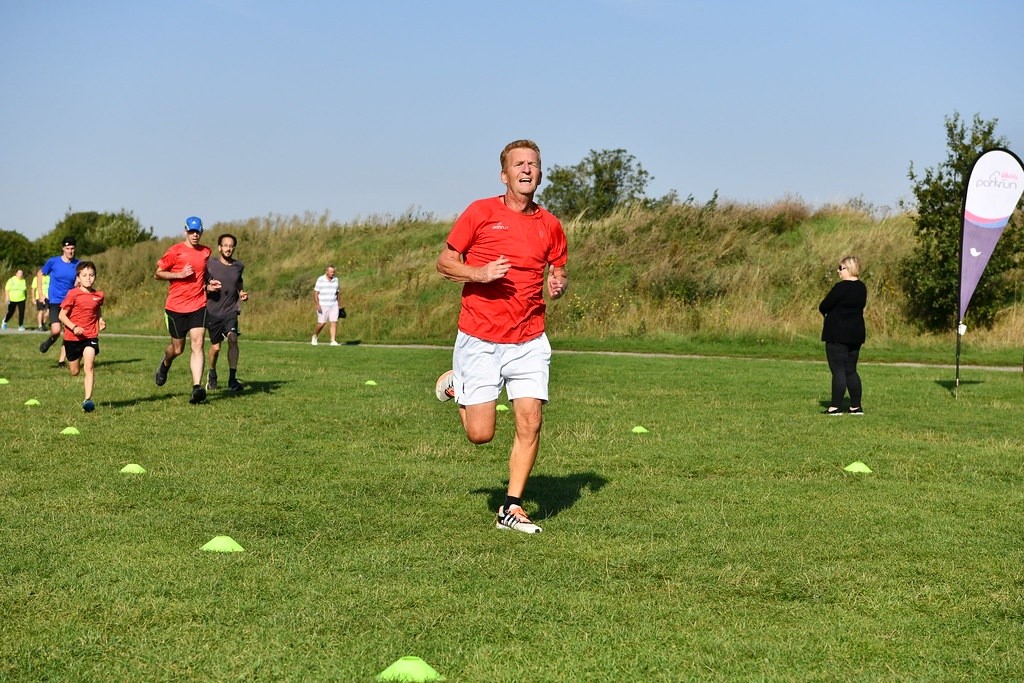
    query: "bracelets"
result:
[205,285,208,291]
[72,326,77,332]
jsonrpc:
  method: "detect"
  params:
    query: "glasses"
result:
[839,265,847,270]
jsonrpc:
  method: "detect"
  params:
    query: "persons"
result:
[58,261,106,412]
[155,216,223,405]
[436,140,568,535]
[204,233,249,391]
[819,256,867,417]
[31,267,51,330]
[2,268,27,332]
[311,266,341,346]
[37,236,80,367]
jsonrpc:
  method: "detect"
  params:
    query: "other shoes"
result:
[43,323,47,331]
[19,326,25,331]
[1,320,7,329]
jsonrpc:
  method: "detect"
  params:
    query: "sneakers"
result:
[497,504,542,534]
[205,373,218,390]
[82,398,94,412]
[312,334,318,345]
[228,379,243,391]
[329,340,341,346]
[822,408,843,415]
[39,338,54,352]
[435,370,455,402]
[155,358,171,386]
[846,407,863,414]
[188,387,207,404]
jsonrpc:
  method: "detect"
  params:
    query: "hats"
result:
[185,216,202,232]
